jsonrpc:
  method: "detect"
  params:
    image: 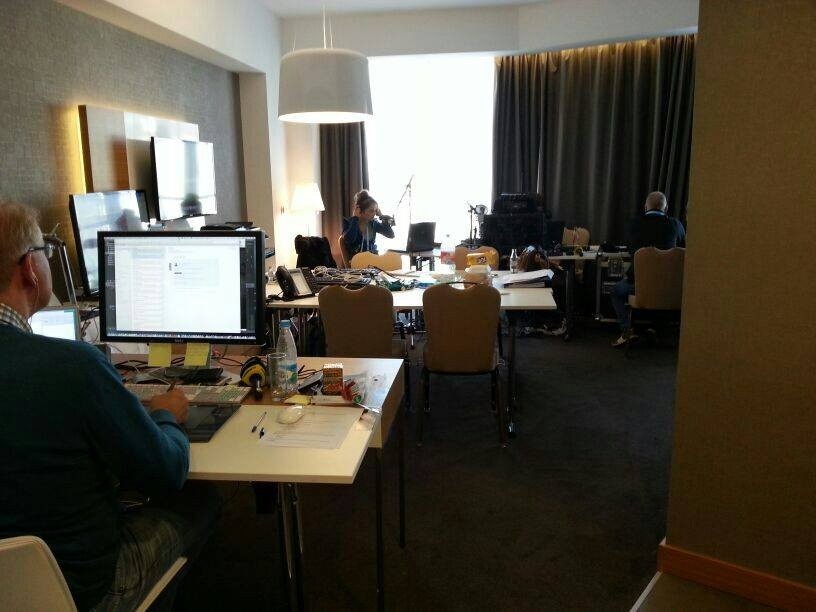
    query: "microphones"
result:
[239,355,269,399]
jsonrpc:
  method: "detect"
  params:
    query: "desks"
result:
[407,244,602,346]
[107,354,406,612]
[265,269,558,437]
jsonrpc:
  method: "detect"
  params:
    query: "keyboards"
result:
[124,383,251,405]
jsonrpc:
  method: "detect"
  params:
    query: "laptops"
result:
[389,222,436,254]
[95,230,266,381]
[27,305,81,341]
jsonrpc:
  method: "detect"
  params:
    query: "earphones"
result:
[32,272,37,280]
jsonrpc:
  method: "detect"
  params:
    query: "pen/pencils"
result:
[167,379,178,391]
[252,411,267,433]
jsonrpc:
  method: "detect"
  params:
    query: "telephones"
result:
[274,266,318,297]
[598,240,620,253]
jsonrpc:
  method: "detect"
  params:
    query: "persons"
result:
[342,190,395,259]
[611,191,685,347]
[0,201,227,611]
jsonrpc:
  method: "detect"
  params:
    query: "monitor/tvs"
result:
[150,136,218,220]
[66,187,145,297]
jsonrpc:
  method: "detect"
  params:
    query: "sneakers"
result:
[639,327,654,343]
[612,326,638,345]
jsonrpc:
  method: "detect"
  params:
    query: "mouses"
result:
[278,406,304,424]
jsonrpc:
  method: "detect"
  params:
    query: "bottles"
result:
[510,249,518,274]
[275,320,299,392]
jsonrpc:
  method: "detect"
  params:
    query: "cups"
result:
[267,353,287,402]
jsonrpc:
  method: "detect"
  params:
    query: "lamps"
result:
[278,0,373,124]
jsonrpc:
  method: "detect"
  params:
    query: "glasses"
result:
[18,244,53,265]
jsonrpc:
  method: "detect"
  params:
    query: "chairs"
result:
[418,281,508,449]
[628,247,686,349]
[337,235,351,270]
[1,535,188,612]
[455,244,502,356]
[319,284,413,417]
[350,251,405,338]
